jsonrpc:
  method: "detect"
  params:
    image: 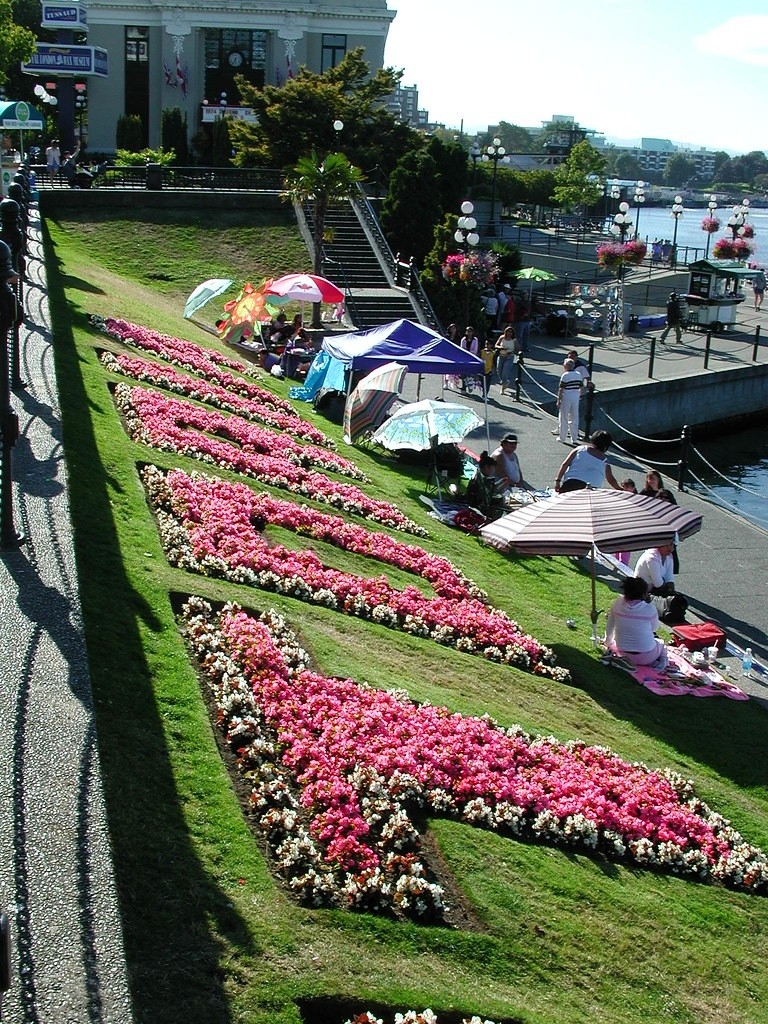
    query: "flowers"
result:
[701,215,758,260]
[596,240,647,275]
[441,249,502,289]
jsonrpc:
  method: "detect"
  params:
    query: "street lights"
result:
[482,138,510,237]
[634,180,645,242]
[34,84,58,129]
[333,120,344,156]
[724,199,752,295]
[705,195,718,260]
[610,178,621,226]
[454,201,480,331]
[669,195,684,269]
[470,142,481,201]
[609,201,633,287]
[76,95,87,147]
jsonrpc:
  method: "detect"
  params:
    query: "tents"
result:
[322,319,491,456]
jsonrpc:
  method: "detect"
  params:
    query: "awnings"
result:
[716,267,764,279]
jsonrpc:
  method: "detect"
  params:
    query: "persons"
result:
[651,238,677,267]
[752,268,768,312]
[265,314,313,350]
[443,283,689,671]
[0,138,98,189]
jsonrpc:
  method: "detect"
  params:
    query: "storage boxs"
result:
[672,622,727,653]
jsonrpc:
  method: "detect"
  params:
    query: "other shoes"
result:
[443,385,448,389]
[757,306,760,311]
[660,340,666,344]
[500,391,504,395]
[556,437,565,442]
[572,441,578,444]
[553,430,559,434]
[676,340,683,343]
[483,398,489,402]
[529,349,532,352]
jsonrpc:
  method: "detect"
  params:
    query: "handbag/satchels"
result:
[479,303,486,313]
[493,354,498,367]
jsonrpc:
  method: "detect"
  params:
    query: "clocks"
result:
[227,50,246,69]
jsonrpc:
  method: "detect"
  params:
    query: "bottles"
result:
[741,648,753,677]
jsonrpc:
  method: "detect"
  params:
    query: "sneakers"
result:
[611,656,638,673]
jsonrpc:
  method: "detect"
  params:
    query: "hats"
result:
[504,284,512,289]
[503,433,519,443]
[521,290,528,294]
[670,292,678,297]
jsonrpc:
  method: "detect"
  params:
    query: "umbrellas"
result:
[183,279,232,319]
[342,361,408,445]
[217,278,273,349]
[506,267,559,314]
[480,483,706,648]
[375,399,485,503]
[264,272,346,328]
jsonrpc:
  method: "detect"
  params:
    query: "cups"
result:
[512,486,518,493]
[707,646,719,663]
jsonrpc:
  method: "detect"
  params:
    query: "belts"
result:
[624,652,642,654]
